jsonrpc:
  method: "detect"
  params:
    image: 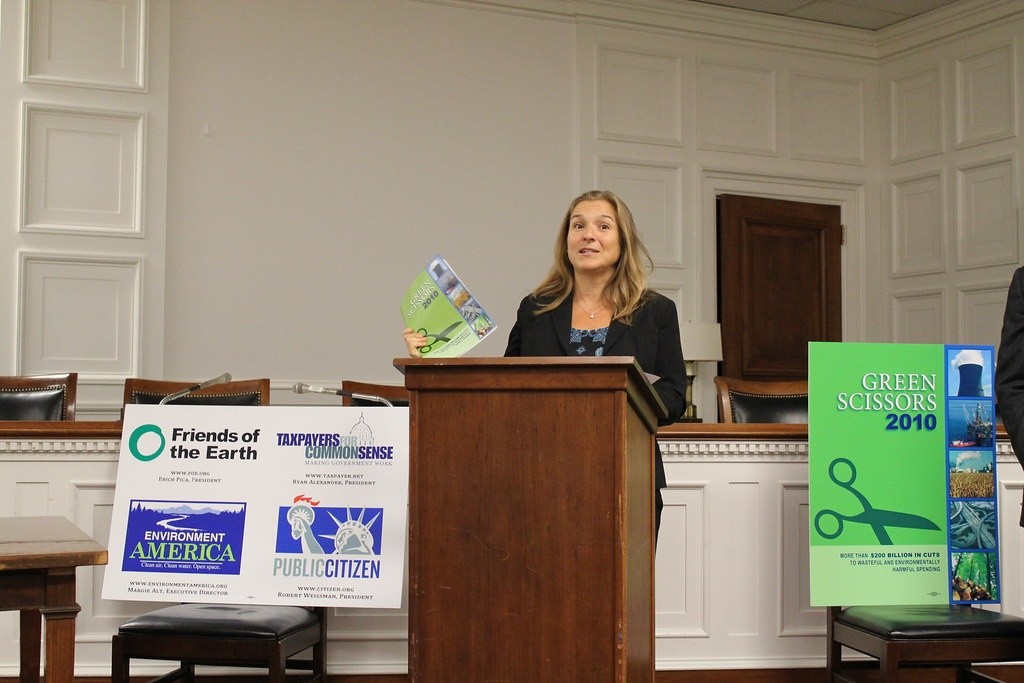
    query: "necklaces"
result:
[573,292,603,318]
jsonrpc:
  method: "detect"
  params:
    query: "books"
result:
[399,255,497,357]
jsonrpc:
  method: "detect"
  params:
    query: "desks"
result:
[0,515,108,683]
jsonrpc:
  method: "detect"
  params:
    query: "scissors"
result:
[415,319,463,354]
[814,456,943,546]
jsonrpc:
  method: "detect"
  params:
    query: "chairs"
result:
[123,378,270,407]
[0,373,78,421]
[826,606,1024,683]
[111,608,328,683]
[342,380,409,407]
[714,376,808,423]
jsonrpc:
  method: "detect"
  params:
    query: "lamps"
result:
[678,321,723,423]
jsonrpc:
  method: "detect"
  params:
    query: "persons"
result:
[403,190,687,553]
[994,266,1023,526]
